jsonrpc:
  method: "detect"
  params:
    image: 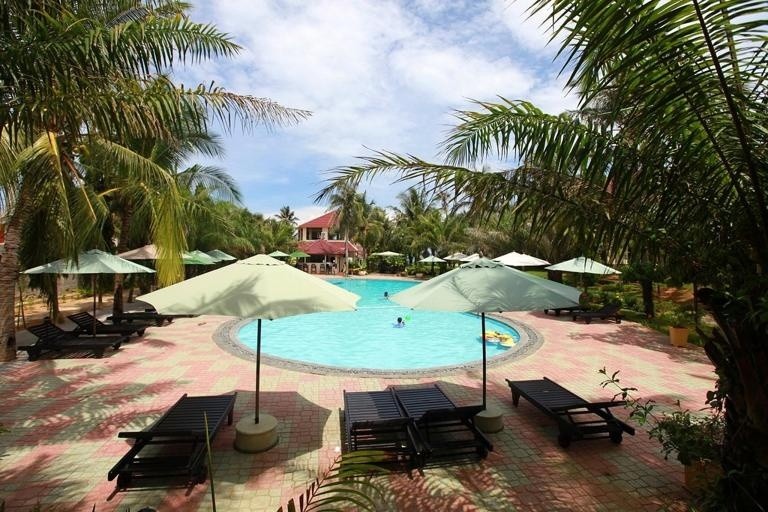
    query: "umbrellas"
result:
[372,251,622,410]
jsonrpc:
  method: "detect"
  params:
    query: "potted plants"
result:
[669,312,690,347]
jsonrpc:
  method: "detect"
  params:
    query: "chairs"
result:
[544,297,626,324]
[15,308,201,361]
[341,375,635,478]
[107,390,238,486]
